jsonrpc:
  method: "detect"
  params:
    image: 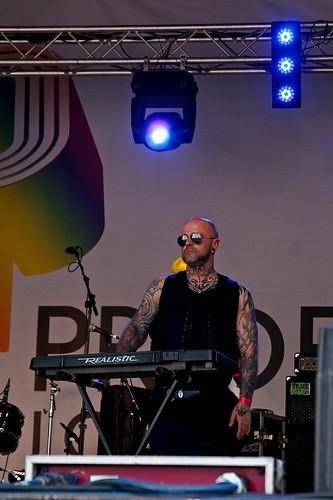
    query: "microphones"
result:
[65,246,79,254]
[2,382,10,404]
[88,324,120,340]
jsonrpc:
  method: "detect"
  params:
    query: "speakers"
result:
[286,375,317,495]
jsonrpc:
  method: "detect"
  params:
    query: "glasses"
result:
[177,232,216,247]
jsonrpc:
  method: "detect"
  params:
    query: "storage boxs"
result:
[237,411,286,455]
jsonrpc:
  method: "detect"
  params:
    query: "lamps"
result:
[271,20,301,110]
[130,71,198,152]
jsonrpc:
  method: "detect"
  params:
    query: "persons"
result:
[116,217,260,456]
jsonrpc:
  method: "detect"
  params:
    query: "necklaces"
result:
[185,270,219,295]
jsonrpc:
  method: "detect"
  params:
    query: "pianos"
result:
[28,351,240,378]
